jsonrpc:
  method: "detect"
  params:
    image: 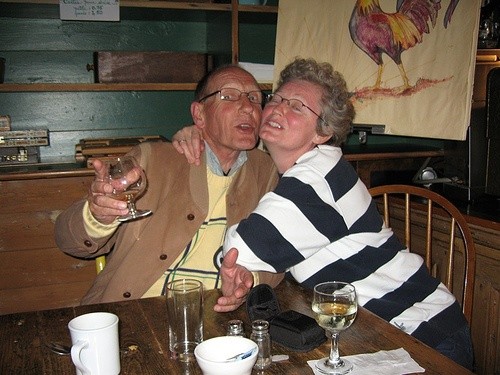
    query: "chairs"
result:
[369,184,475,328]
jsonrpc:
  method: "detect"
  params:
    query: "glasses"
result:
[199,87,265,104]
[267,91,326,123]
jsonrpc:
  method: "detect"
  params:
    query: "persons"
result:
[55,65,284,312]
[173,58,475,368]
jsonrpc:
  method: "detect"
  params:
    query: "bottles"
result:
[250,319,272,371]
[226,319,247,338]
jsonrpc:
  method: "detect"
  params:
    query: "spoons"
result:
[47,342,131,356]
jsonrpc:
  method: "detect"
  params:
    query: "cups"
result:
[68,311,122,375]
[164,279,204,364]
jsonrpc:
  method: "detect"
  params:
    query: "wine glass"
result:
[102,156,152,222]
[311,281,359,375]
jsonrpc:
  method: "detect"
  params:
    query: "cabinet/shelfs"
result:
[0,0,277,91]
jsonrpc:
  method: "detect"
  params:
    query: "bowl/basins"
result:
[194,336,259,375]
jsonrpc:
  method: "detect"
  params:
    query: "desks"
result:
[0,277,474,375]
[341,143,448,189]
[0,163,117,314]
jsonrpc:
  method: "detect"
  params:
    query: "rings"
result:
[178,139,186,142]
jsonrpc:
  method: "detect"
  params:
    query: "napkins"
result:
[307,347,426,375]
